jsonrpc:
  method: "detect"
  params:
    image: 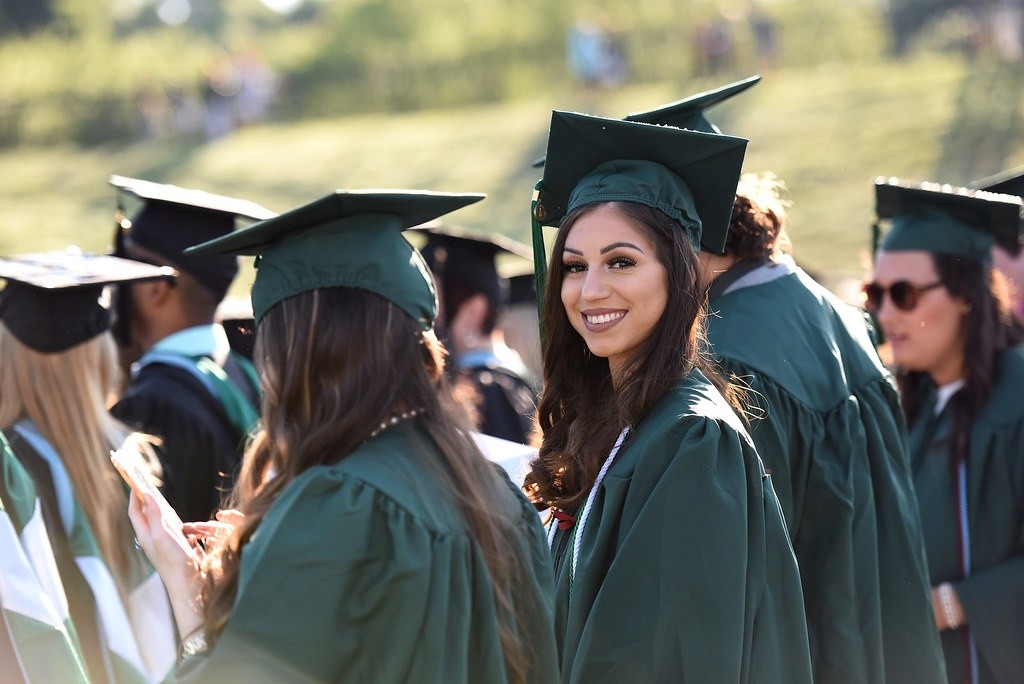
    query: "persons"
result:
[0,74,1024,684]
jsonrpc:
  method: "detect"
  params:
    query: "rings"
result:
[134,537,144,552]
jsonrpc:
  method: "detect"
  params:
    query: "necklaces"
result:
[362,406,427,446]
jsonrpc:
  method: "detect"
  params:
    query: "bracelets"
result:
[182,630,207,657]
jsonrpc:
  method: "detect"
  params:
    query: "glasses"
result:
[862,278,941,313]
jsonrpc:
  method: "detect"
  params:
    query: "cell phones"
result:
[111,449,193,560]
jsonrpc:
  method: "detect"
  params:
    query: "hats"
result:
[530,73,763,365]
[406,224,533,282]
[0,252,180,354]
[869,167,1024,269]
[184,190,489,332]
[108,173,280,303]
